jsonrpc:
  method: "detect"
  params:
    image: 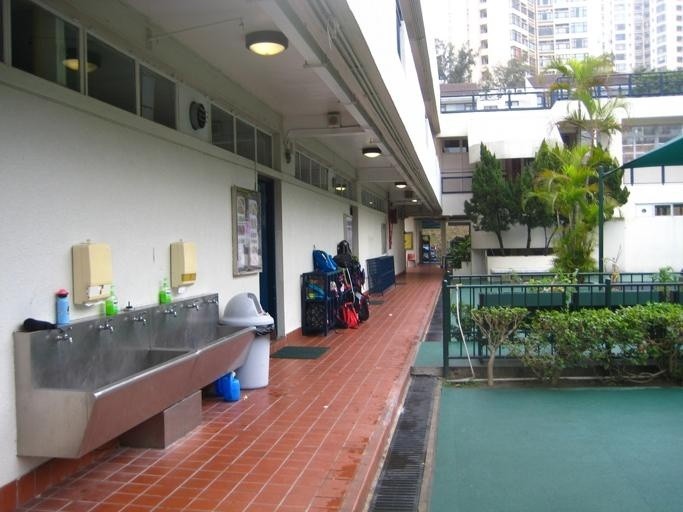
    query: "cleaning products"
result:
[57,289,70,325]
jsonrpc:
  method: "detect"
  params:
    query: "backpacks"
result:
[308,240,370,330]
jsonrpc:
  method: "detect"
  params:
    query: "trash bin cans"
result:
[218,292,275,389]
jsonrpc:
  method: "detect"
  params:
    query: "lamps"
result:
[63,51,101,72]
[363,147,381,158]
[335,185,346,191]
[245,31,288,56]
[395,182,406,189]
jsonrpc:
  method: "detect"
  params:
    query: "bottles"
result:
[56,288,71,325]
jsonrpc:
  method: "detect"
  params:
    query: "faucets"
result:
[98,318,113,333]
[130,311,148,326]
[55,326,73,343]
[163,304,177,317]
[209,295,218,306]
[188,300,200,311]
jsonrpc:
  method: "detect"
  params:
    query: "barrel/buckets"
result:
[223,370,241,400]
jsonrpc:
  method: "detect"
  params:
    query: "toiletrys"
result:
[106,290,120,315]
[160,279,171,303]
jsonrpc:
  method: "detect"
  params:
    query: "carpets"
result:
[369,301,384,305]
[270,346,329,360]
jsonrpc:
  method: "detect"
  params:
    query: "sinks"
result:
[33,349,190,395]
[149,321,254,357]
